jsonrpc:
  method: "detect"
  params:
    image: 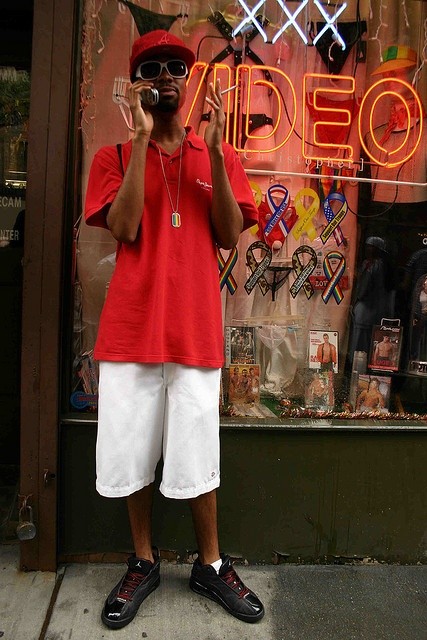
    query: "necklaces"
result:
[151,135,192,228]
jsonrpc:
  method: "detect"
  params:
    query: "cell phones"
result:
[141,88,159,105]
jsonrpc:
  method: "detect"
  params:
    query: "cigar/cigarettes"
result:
[217,83,240,99]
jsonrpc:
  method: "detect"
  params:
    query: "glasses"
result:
[135,58,191,80]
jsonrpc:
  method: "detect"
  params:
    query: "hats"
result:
[130,29,196,84]
[370,45,419,76]
[366,236,391,255]
[386,76,423,132]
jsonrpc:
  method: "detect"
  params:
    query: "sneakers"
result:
[189,552,264,623]
[101,546,161,629]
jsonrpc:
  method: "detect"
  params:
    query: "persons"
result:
[317,332,337,371]
[229,366,240,392]
[356,376,386,412]
[235,369,249,402]
[306,371,326,403]
[247,366,259,395]
[81,30,262,632]
[372,333,398,367]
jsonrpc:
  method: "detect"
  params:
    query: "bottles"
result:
[349,351,368,413]
